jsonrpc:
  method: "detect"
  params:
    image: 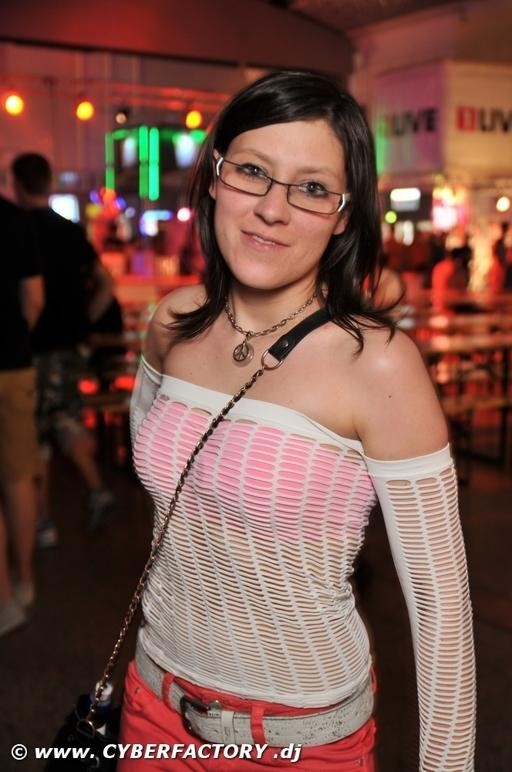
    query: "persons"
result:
[10,154,114,549]
[0,183,48,636]
[111,70,478,772]
[100,220,512,300]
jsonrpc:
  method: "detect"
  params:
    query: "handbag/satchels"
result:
[44,694,122,772]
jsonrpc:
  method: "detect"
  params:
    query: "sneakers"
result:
[37,485,118,549]
[0,584,37,638]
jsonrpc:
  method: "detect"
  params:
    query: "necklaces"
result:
[222,291,320,368]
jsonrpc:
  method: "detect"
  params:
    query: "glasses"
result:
[210,147,352,218]
